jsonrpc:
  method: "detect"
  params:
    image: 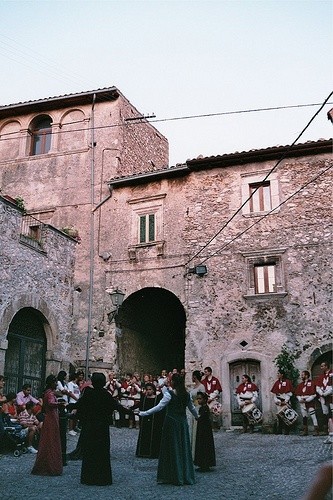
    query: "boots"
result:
[249,425,253,434]
[240,425,247,433]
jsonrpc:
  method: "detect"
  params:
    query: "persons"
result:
[235,374,259,434]
[300,460,333,500]
[0,368,186,453]
[270,369,295,435]
[126,383,166,458]
[200,366,222,432]
[186,371,216,471]
[65,382,98,461]
[65,372,138,486]
[295,371,320,436]
[30,374,65,476]
[316,362,333,436]
[138,372,202,486]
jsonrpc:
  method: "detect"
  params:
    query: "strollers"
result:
[0,408,28,457]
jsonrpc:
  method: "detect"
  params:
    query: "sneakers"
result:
[27,445,38,454]
[323,435,333,444]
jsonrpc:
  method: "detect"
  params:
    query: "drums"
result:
[121,397,135,406]
[276,405,299,425]
[209,400,224,417]
[241,403,263,424]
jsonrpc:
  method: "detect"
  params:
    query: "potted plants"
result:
[273,344,302,433]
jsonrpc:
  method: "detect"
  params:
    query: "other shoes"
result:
[69,430,78,436]
[214,428,219,432]
[120,421,128,426]
[116,422,121,428]
[299,431,307,435]
[129,424,132,428]
[73,427,79,431]
[77,428,82,431]
[136,423,139,429]
[319,430,328,436]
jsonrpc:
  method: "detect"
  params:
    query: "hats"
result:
[77,371,84,378]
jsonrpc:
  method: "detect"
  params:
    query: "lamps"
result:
[195,264,208,277]
[107,288,126,325]
